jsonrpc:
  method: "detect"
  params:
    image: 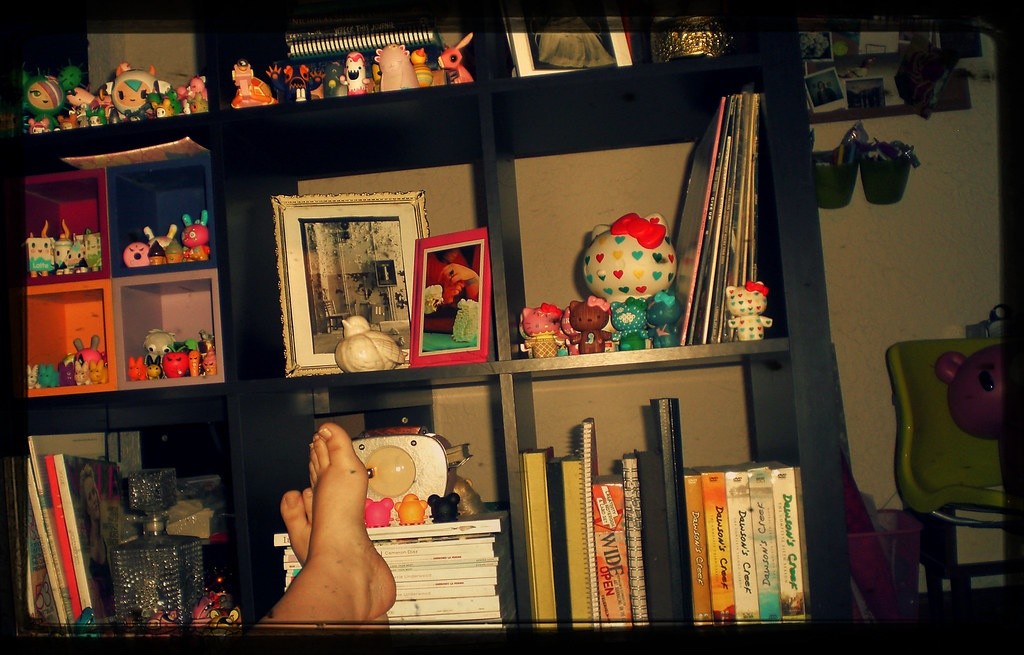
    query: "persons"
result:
[815,81,837,106]
[144,329,176,365]
[78,465,116,617]
[265,422,397,622]
[799,32,831,59]
[424,245,480,331]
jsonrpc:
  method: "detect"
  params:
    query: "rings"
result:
[450,271,453,277]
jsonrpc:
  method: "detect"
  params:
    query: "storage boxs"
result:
[848,507,923,624]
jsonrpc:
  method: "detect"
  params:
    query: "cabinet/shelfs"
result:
[14,13,859,638]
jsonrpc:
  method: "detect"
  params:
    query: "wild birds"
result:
[334,313,406,373]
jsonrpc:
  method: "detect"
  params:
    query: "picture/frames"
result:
[505,15,633,79]
[269,190,431,379]
[409,225,492,369]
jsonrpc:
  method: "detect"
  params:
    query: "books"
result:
[518,394,808,624]
[5,431,142,624]
[273,512,515,625]
[669,92,760,346]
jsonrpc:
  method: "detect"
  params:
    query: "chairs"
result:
[885,336,1011,624]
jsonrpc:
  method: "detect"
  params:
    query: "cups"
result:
[861,155,913,205]
[814,150,859,209]
